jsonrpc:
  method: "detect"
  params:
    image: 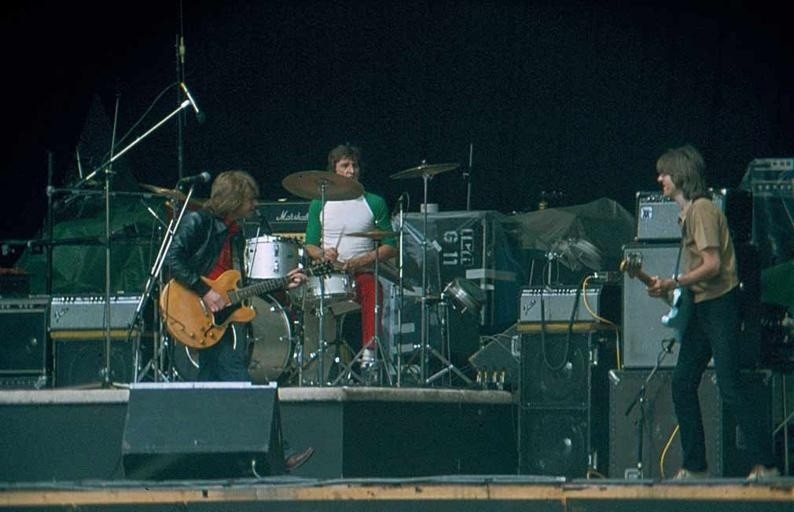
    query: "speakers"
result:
[469,321,521,391]
[521,330,621,480]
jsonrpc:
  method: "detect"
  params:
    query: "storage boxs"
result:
[606,368,775,481]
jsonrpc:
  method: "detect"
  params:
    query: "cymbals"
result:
[138,183,202,207]
[281,169,363,200]
[390,163,460,180]
[345,229,410,240]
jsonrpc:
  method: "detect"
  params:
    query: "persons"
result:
[304,144,397,386]
[645,145,783,480]
[165,169,314,472]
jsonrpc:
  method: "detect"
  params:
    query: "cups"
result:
[420,204,439,214]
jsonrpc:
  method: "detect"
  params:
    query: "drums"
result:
[448,279,487,317]
[295,274,356,304]
[244,236,298,280]
[247,293,295,384]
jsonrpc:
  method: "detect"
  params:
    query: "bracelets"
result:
[673,275,680,285]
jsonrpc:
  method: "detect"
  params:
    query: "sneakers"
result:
[360,346,381,369]
[745,461,782,481]
[673,466,713,481]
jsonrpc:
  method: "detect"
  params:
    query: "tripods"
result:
[77,101,189,389]
[125,185,474,390]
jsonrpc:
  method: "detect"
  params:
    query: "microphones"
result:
[179,172,211,185]
[181,82,206,124]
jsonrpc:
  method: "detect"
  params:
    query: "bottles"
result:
[539,190,564,211]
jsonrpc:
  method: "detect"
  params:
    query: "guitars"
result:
[159,258,334,350]
[619,253,695,343]
